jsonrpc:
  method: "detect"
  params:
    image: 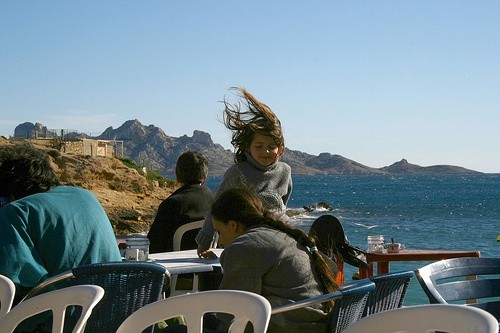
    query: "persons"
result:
[210,187,332,333]
[196,86,293,260]
[0,142,121,318]
[146,152,215,255]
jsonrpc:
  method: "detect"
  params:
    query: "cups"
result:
[387,243,401,253]
[125,237,150,260]
[367,235,385,253]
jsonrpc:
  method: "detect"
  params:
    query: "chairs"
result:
[0,220,500,333]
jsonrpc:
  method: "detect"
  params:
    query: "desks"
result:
[362,248,481,307]
[146,248,228,295]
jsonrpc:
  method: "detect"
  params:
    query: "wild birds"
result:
[351,222,383,230]
